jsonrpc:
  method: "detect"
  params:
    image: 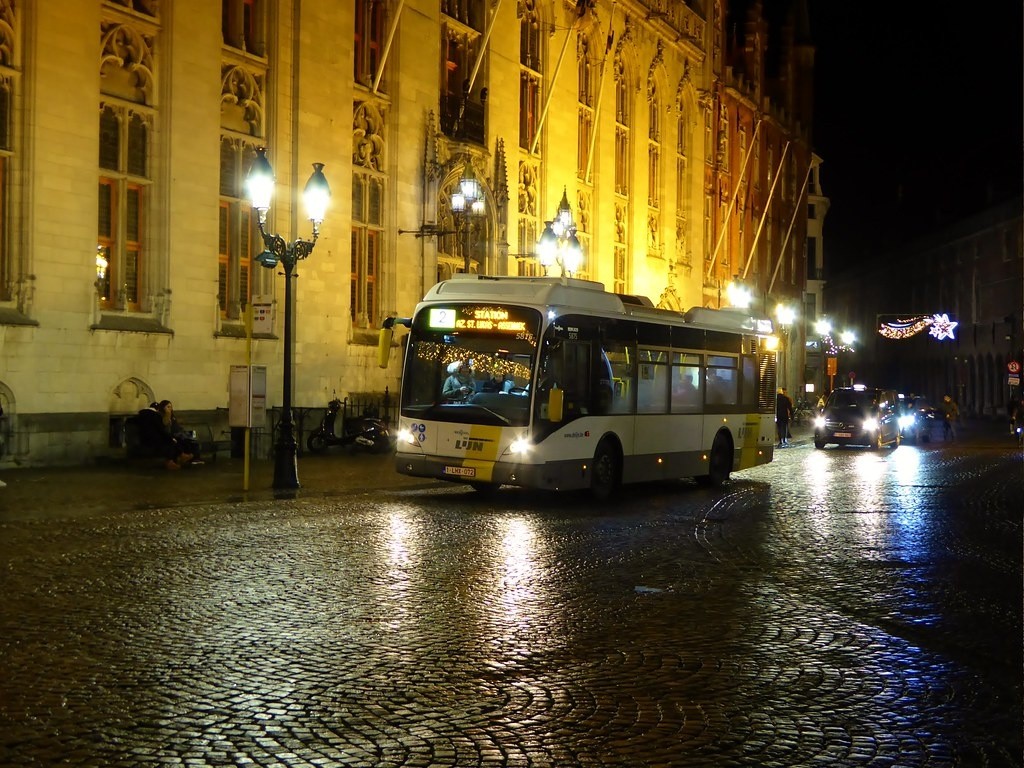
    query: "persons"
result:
[816,393,828,411]
[776,387,794,449]
[441,362,476,404]
[706,367,729,398]
[483,370,507,393]
[943,394,961,440]
[1009,394,1024,444]
[522,364,560,400]
[133,402,194,471]
[672,373,698,405]
[158,400,206,464]
[783,388,794,438]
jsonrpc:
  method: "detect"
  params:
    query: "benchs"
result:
[126,418,235,464]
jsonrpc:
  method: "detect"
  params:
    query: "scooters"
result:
[307,389,390,455]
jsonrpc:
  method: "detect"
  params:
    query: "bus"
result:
[375,273,779,504]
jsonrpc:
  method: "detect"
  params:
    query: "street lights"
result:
[776,303,795,393]
[245,147,332,494]
[452,156,488,277]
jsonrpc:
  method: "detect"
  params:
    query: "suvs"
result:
[814,384,900,450]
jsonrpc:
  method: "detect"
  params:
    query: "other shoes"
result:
[181,452,194,462]
[165,460,181,469]
[191,458,205,465]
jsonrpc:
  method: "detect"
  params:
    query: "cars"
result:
[897,393,952,445]
